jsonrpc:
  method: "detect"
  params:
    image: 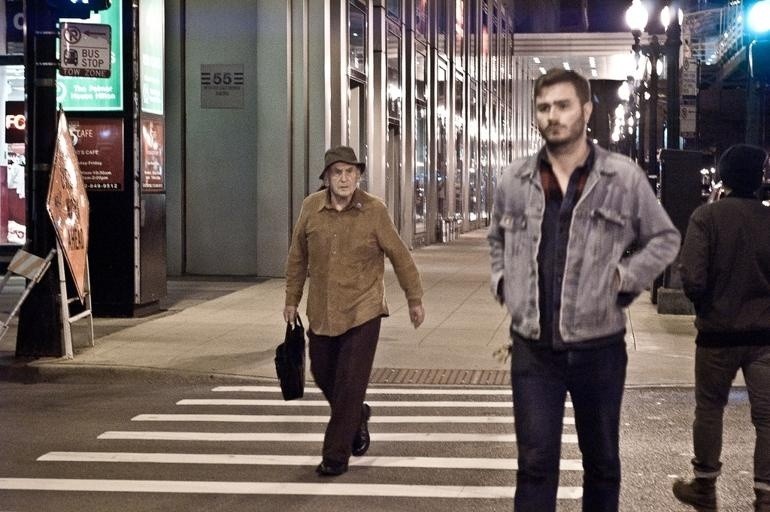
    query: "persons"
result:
[674,143,769,511]
[283,145,426,476]
[485,67,682,511]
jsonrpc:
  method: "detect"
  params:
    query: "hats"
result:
[319,147,366,180]
[719,144,768,193]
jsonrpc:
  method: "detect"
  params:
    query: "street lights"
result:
[612,0,683,196]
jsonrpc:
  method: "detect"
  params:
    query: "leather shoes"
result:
[352,404,370,456]
[316,460,348,475]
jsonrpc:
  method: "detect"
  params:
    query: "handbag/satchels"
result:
[275,326,306,400]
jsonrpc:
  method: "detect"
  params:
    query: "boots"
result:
[751,482,770,511]
[673,465,722,511]
